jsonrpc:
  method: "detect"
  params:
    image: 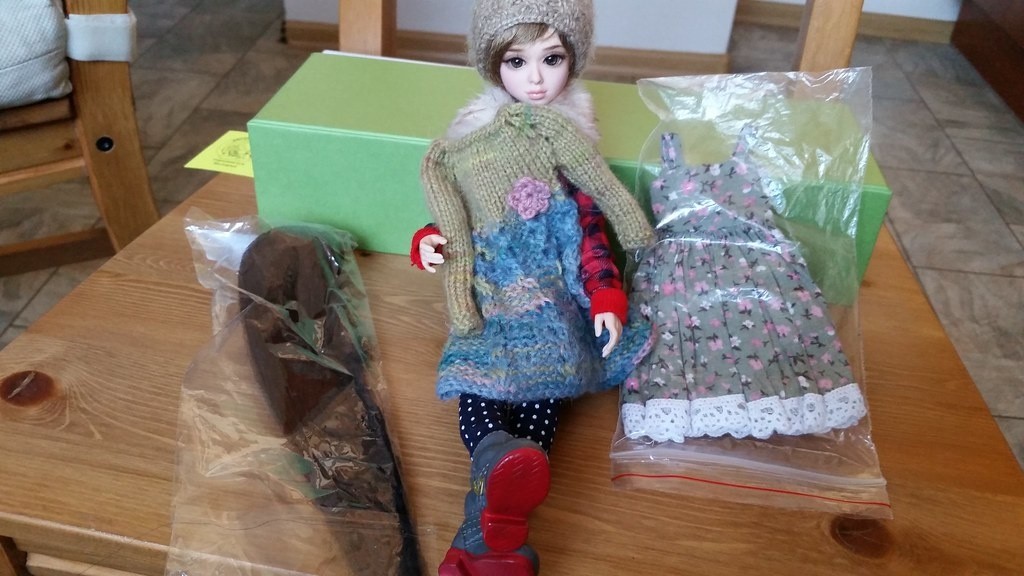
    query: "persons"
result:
[410,1,628,575]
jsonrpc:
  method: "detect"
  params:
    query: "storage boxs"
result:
[247,50,891,303]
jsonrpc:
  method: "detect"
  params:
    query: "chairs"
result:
[0,0,1024,576]
[0,0,163,279]
